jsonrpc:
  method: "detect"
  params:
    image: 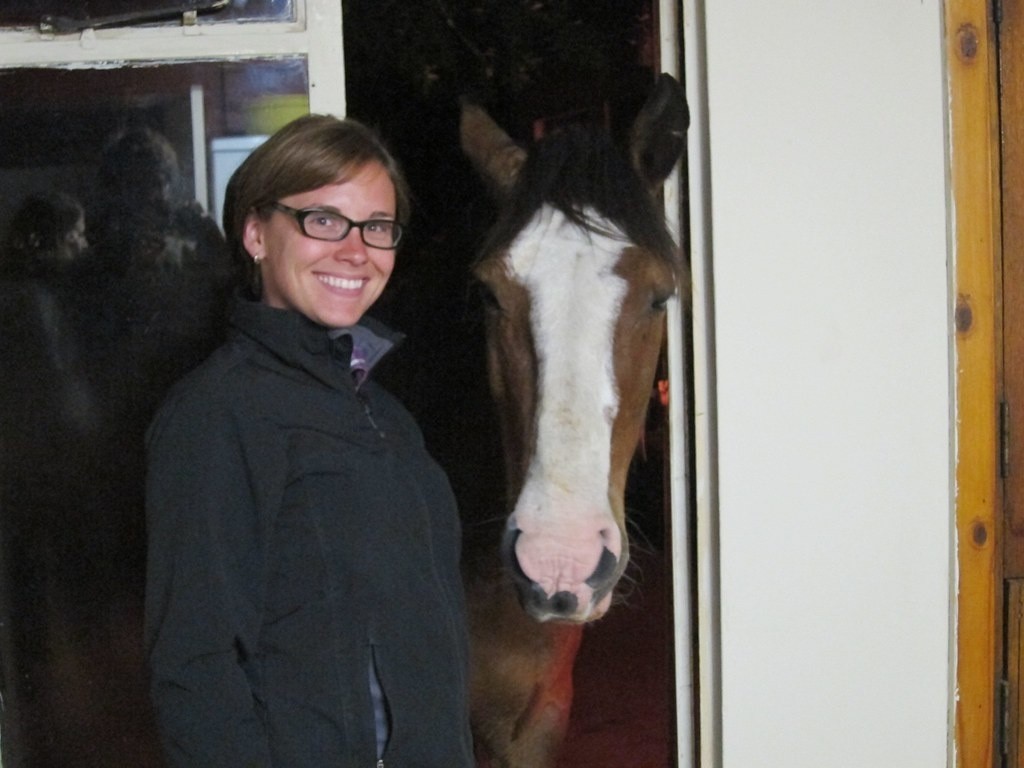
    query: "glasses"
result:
[270,199,405,251]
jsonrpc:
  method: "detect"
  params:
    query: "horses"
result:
[371,71,692,768]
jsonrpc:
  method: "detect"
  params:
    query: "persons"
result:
[141,113,479,768]
